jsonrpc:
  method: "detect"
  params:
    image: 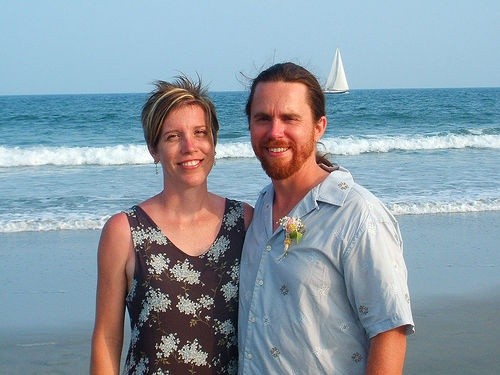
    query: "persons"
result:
[237,62,414,375]
[90,70,254,375]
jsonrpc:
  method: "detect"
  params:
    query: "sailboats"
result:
[321,47,349,94]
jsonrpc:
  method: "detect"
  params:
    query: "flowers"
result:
[274,215,307,264]
